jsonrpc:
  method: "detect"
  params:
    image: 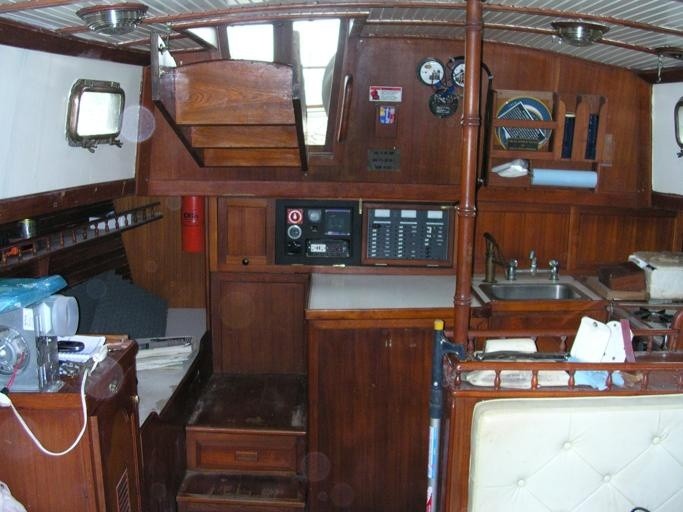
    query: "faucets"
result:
[528,249,537,274]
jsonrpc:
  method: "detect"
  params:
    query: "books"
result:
[56,334,105,364]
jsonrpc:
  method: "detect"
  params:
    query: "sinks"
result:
[471,273,603,306]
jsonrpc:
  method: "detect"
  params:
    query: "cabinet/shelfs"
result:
[1,333,147,512]
[209,194,275,272]
[331,38,468,185]
[303,310,436,511]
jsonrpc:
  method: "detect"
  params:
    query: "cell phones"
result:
[58,341,84,352]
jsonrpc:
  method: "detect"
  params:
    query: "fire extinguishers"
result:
[181,196,203,254]
